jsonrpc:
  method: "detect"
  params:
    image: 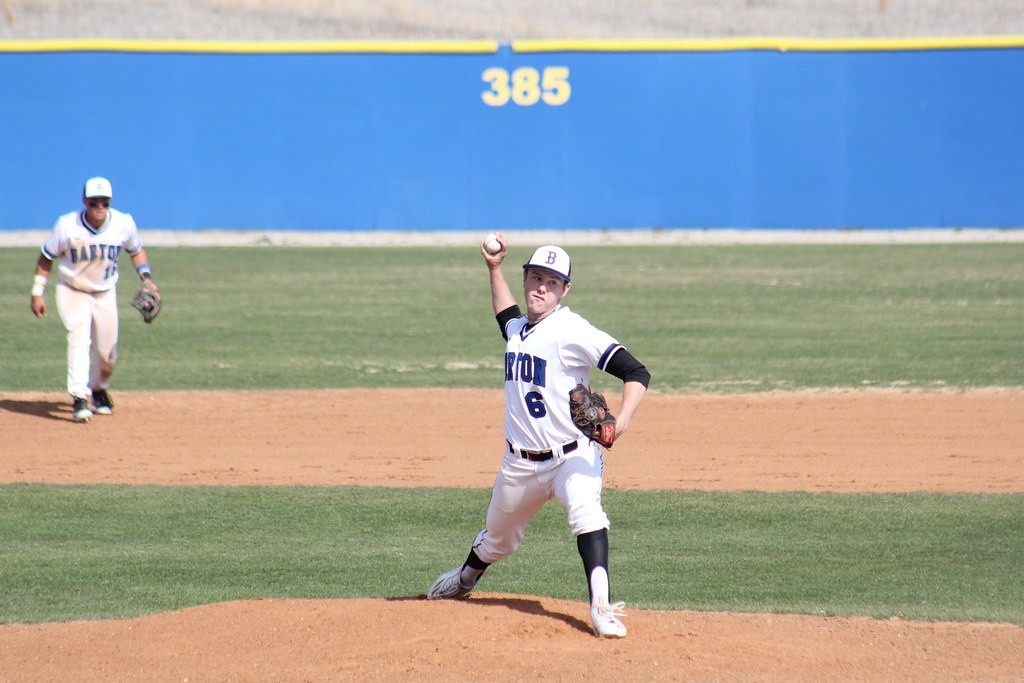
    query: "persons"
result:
[30,176,162,422]
[426,231,651,638]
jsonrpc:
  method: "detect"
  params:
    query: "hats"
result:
[85,177,112,197]
[523,245,571,283]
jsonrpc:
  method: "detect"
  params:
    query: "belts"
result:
[507,440,578,461]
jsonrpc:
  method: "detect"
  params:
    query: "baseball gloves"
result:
[568,383,616,449]
[130,282,164,325]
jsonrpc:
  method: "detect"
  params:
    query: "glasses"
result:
[87,200,109,207]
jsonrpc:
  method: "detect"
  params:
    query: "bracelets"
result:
[137,264,151,282]
[31,274,47,296]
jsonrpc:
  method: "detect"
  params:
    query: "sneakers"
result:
[73,398,92,423]
[92,389,113,415]
[426,565,475,600]
[589,596,627,637]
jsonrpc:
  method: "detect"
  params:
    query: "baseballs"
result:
[483,232,502,254]
